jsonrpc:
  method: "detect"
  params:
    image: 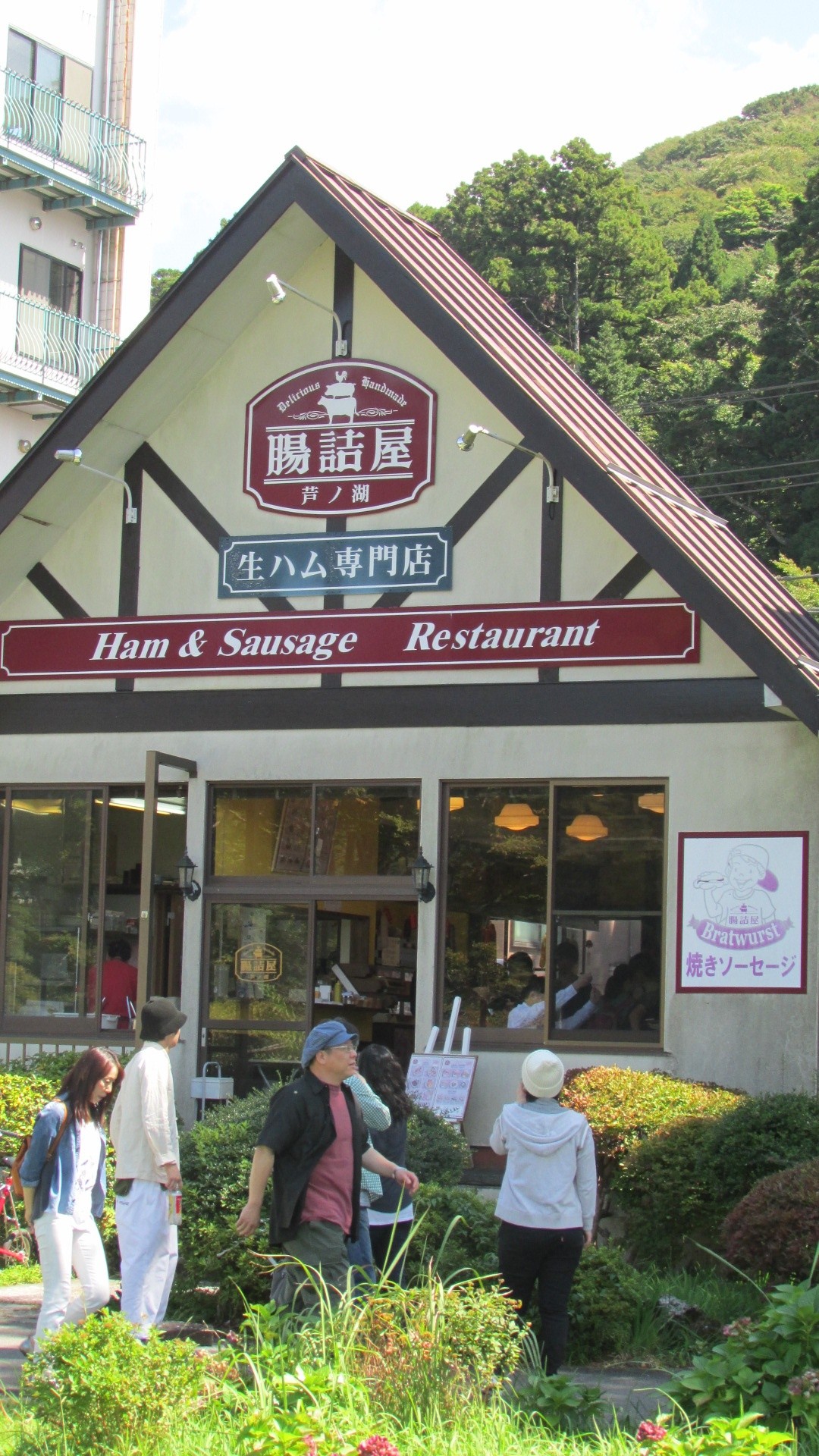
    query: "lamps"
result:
[406,846,436,905]
[175,845,202,902]
[418,787,663,846]
[53,447,137,524]
[457,429,559,519]
[266,273,349,358]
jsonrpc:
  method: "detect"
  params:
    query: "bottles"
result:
[334,980,341,1002]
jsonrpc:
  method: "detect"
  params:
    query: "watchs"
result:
[391,1166,407,1180]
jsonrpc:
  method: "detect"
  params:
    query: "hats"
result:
[302,1021,358,1068]
[139,999,187,1041]
[522,1049,564,1098]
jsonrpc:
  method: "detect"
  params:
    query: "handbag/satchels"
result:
[11,1134,37,1199]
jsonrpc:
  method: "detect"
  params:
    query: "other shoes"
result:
[19,1338,34,1356]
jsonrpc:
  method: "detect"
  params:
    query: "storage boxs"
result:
[53,1012,120,1030]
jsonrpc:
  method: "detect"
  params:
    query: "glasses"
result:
[322,1043,356,1052]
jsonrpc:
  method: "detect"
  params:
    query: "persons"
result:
[474,939,663,1047]
[16,1046,126,1362]
[232,1021,422,1329]
[106,998,188,1341]
[487,1048,601,1381]
[83,938,140,1032]
[349,1043,416,1293]
[304,1019,393,1307]
